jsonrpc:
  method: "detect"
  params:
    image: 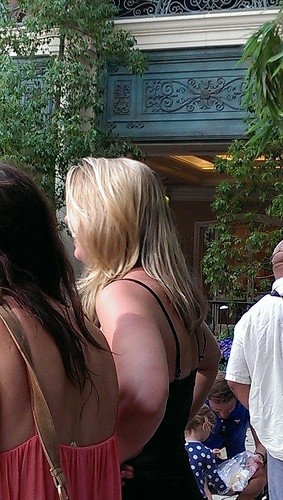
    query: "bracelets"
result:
[253,452,266,463]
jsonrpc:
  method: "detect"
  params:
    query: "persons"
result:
[201,371,269,500]
[0,162,124,499]
[63,156,221,500]
[184,403,236,500]
[225,238,283,500]
